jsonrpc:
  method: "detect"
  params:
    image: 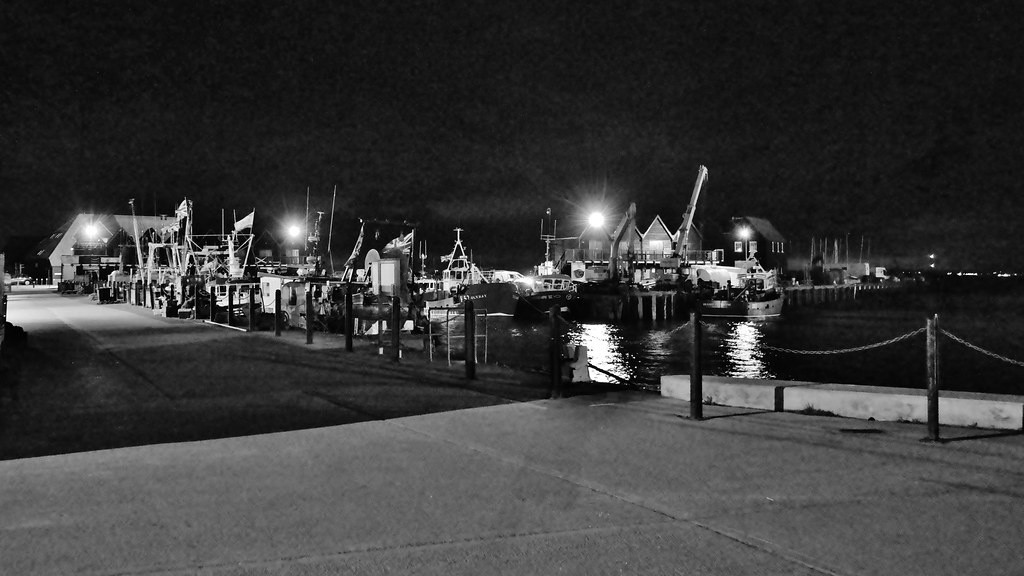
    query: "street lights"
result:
[740,228,751,261]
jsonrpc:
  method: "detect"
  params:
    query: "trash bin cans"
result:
[98,287,112,302]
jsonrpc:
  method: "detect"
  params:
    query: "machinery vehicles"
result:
[585,202,643,289]
[658,165,716,291]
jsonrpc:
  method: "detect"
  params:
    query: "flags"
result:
[382,233,412,257]
[173,201,189,220]
[234,208,255,234]
[344,225,364,269]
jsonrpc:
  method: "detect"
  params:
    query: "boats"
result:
[111,199,434,353]
[698,249,785,319]
[440,229,524,291]
[524,274,578,317]
